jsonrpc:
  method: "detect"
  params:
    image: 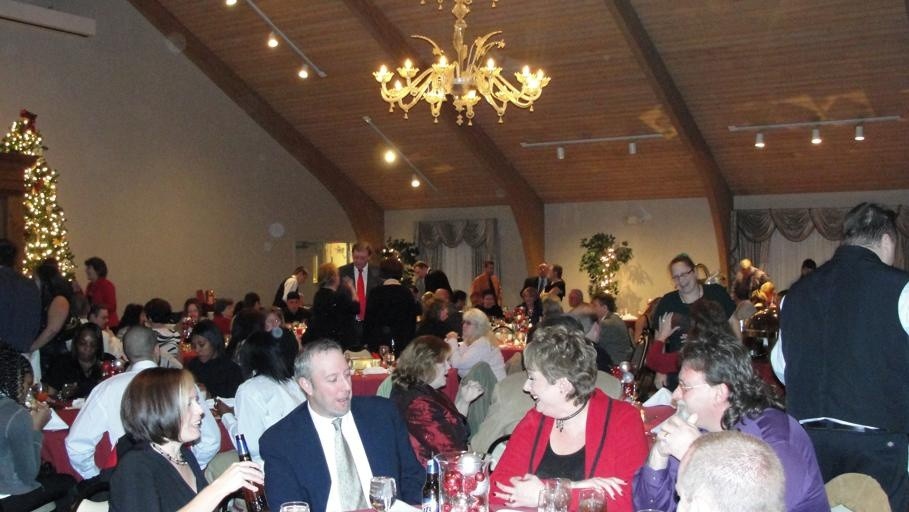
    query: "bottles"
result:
[420,459,439,511]
[485,304,533,353]
[377,334,398,372]
[20,358,126,409]
[233,432,268,511]
[613,359,635,401]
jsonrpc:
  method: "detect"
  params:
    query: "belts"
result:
[805,420,873,433]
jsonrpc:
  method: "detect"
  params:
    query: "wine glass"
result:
[369,474,397,511]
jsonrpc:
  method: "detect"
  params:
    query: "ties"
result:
[489,276,494,290]
[539,277,544,293]
[333,418,364,509]
[356,269,366,319]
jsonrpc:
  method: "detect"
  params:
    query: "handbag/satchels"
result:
[57,312,81,343]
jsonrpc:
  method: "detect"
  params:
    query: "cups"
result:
[538,488,568,511]
[432,449,498,511]
[543,477,572,509]
[577,486,612,511]
[278,500,310,511]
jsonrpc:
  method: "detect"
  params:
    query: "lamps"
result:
[412,173,419,187]
[557,145,565,159]
[811,125,821,144]
[629,140,636,154]
[754,129,766,148]
[373,0,551,126]
[855,122,864,140]
[298,63,309,79]
[268,31,278,47]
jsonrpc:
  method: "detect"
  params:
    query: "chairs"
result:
[823,471,891,512]
[456,362,498,446]
[505,351,523,375]
[376,373,392,398]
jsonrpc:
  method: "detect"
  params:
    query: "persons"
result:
[0,202,909,512]
